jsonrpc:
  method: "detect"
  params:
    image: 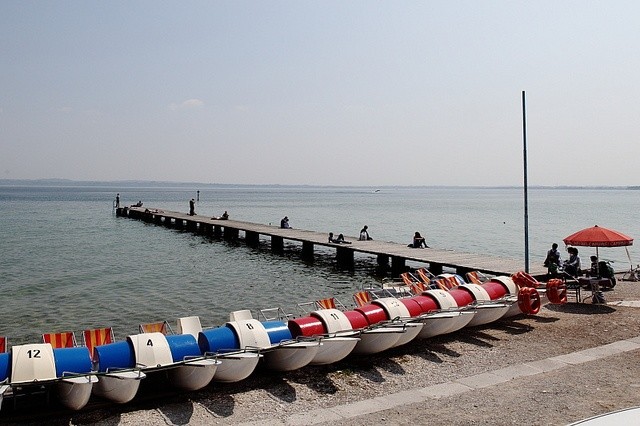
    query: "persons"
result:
[328,232,352,244]
[281,216,288,228]
[563,248,579,278]
[283,219,293,229]
[116,194,119,208]
[190,198,195,216]
[414,232,430,249]
[223,211,229,218]
[131,201,143,207]
[360,225,373,240]
[564,247,574,262]
[583,256,599,289]
[545,243,562,279]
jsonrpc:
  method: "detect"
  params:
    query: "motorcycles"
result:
[598,260,616,289]
[562,260,584,288]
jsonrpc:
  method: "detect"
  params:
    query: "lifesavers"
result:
[518,286,529,314]
[523,287,540,314]
[512,274,526,287]
[546,279,567,304]
[517,271,540,287]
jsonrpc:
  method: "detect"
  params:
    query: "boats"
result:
[0,329,99,412]
[352,284,463,349]
[278,296,407,366]
[467,270,566,321]
[82,319,222,402]
[178,307,322,384]
[414,278,512,347]
[383,270,444,296]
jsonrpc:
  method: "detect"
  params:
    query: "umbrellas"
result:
[562,224,634,261]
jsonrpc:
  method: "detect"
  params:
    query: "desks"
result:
[577,277,612,303]
[561,280,581,304]
